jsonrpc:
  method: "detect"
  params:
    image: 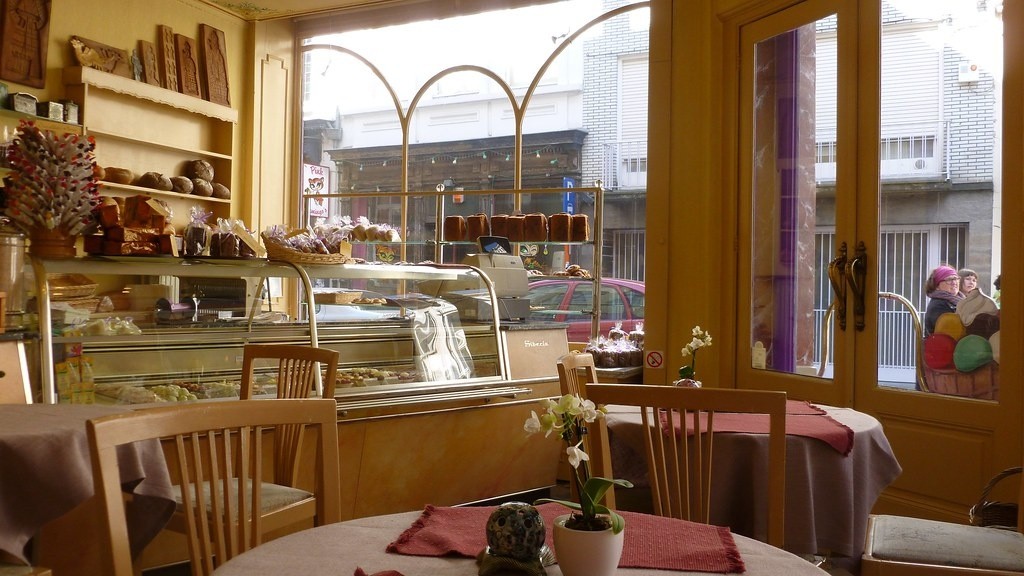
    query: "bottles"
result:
[0,216,28,314]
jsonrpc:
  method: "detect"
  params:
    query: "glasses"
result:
[940,276,960,283]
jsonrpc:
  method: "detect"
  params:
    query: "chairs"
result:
[555,352,598,497]
[133,343,341,576]
[861,515,1024,576]
[585,383,786,551]
[85,398,342,576]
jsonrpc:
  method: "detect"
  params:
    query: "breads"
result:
[188,226,254,257]
[445,214,588,242]
[586,330,643,367]
[85,195,178,256]
[315,225,399,241]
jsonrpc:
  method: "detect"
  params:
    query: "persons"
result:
[925,266,978,336]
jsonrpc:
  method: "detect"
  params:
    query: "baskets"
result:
[261,230,353,266]
[23,271,100,316]
[305,290,363,304]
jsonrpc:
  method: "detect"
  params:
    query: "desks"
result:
[602,404,903,560]
[0,403,177,566]
[210,502,832,576]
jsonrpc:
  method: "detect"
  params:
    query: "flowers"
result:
[523,392,634,535]
[672,325,713,379]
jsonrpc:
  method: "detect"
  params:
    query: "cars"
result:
[525,276,644,342]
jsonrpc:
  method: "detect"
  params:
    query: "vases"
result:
[673,381,702,387]
[552,513,624,576]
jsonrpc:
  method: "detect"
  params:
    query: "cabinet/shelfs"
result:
[62,65,239,237]
[22,252,519,418]
[302,179,605,353]
